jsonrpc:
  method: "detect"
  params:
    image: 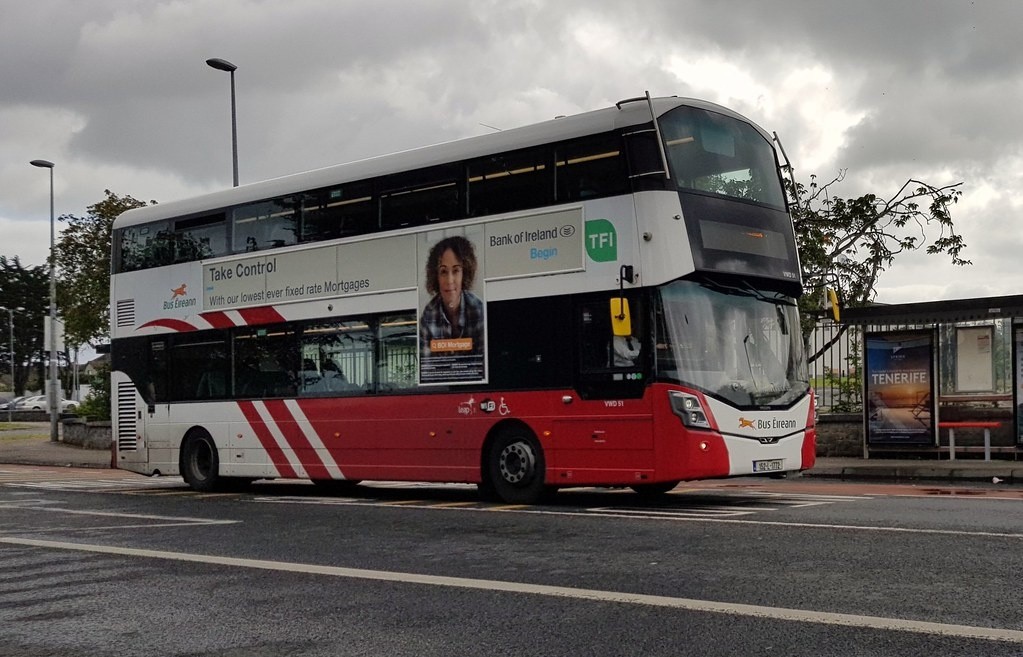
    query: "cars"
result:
[0,396,80,414]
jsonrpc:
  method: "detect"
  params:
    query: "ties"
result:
[625,337,634,351]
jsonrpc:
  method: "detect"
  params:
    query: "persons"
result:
[419,236,486,383]
[606,322,667,381]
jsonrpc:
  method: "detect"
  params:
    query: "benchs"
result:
[938,421,1001,460]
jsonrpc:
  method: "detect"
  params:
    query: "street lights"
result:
[0,305,26,410]
[30,159,60,443]
[205,56,240,188]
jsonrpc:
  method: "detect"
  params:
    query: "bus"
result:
[105,89,844,510]
